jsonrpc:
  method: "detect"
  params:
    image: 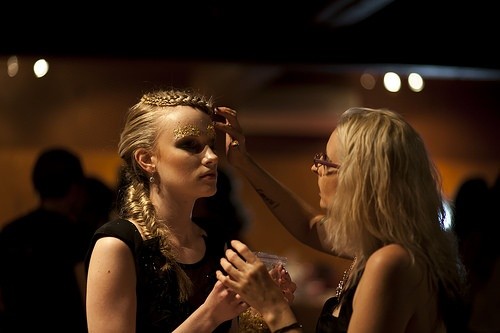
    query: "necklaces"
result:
[335,255,358,304]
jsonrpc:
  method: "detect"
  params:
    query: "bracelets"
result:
[272,319,303,333]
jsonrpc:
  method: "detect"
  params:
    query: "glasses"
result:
[312,151,343,170]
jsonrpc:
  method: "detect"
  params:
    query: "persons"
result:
[84,89,297,333]
[213,106,461,333]
[0,145,88,333]
